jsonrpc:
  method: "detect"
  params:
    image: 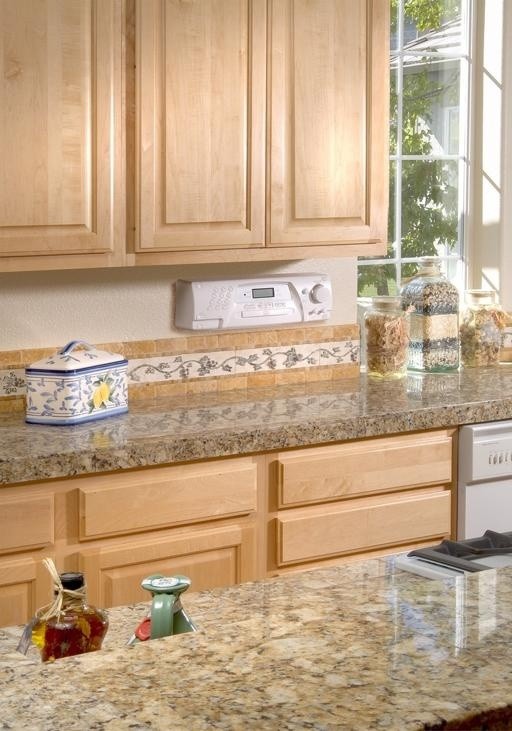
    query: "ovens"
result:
[458,418,511,538]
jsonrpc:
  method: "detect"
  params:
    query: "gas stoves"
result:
[392,531,511,584]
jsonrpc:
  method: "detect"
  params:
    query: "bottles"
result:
[31,570,110,663]
[362,257,512,383]
[128,573,198,646]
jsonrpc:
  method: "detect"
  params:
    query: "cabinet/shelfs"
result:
[263,429,457,573]
[0,2,120,266]
[3,486,68,631]
[131,3,390,262]
[68,453,264,619]
